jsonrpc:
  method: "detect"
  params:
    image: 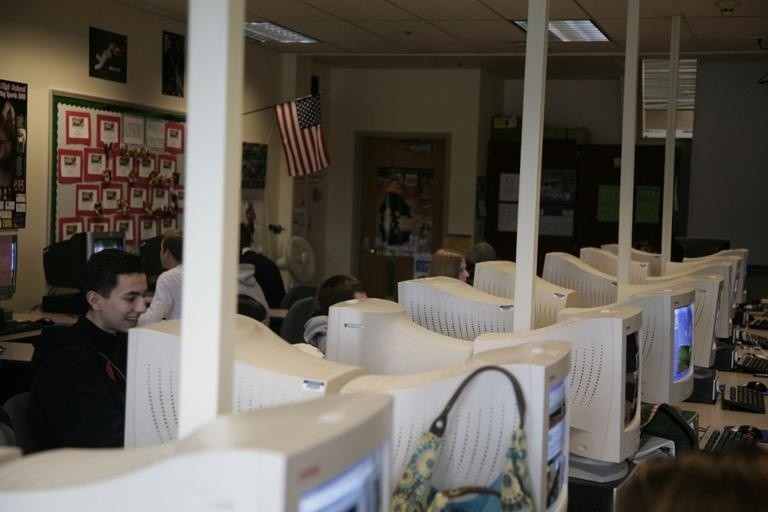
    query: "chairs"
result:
[0,392,33,455]
[273,282,319,345]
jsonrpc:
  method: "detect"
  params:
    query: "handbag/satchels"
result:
[640,402,699,457]
[389,365,533,512]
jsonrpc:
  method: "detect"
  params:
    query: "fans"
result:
[275,236,315,284]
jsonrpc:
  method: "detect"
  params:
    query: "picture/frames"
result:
[88,23,185,99]
[243,143,269,189]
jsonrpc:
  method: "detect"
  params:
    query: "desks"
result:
[0,309,77,409]
[679,312,768,431]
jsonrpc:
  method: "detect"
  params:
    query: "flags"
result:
[275,92,331,178]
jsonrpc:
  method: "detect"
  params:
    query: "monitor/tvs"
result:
[0,225,767,512]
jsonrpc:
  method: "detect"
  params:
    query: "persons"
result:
[241,222,288,308]
[381,171,424,246]
[133,228,185,327]
[427,245,471,283]
[24,247,151,452]
[466,240,498,272]
[303,273,370,355]
[240,247,272,328]
[237,295,268,324]
